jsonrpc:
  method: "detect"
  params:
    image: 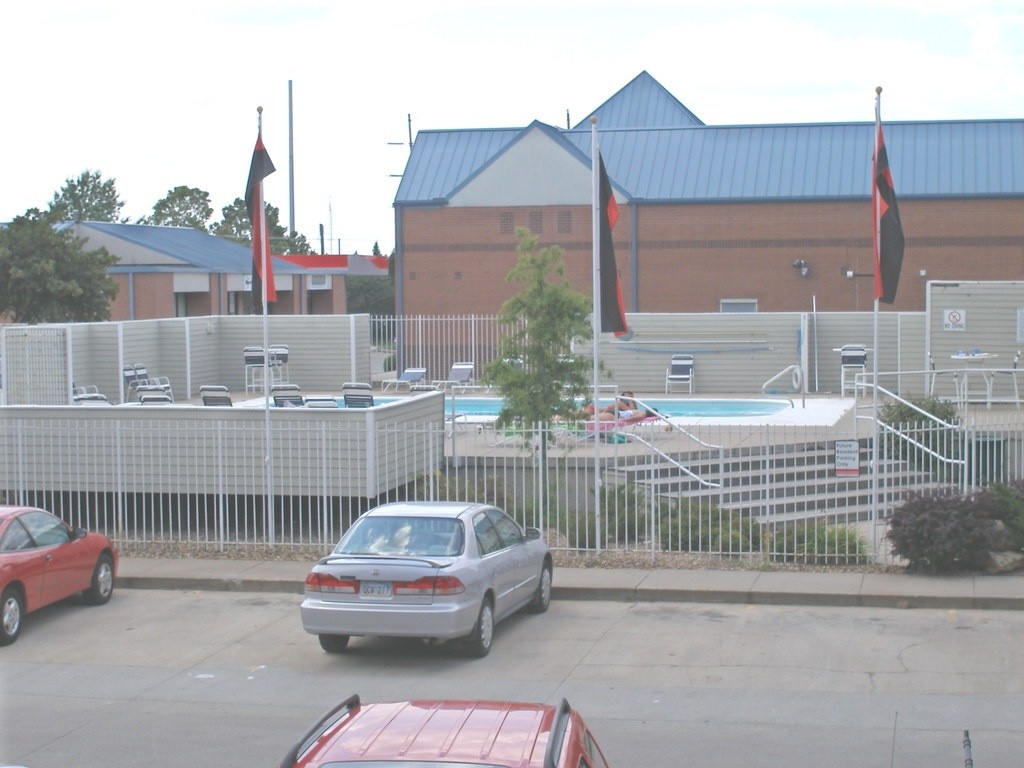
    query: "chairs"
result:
[477,413,671,452]
[72,377,112,405]
[305,395,337,408]
[839,344,867,398]
[199,385,233,407]
[986,349,1021,411]
[123,363,157,403]
[139,396,172,403]
[260,343,289,394]
[431,361,474,393]
[134,363,174,404]
[665,352,695,395]
[244,346,273,397]
[381,367,427,394]
[342,383,374,408]
[270,384,304,408]
[928,352,959,400]
[408,385,467,437]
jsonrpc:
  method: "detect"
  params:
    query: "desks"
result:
[949,353,999,409]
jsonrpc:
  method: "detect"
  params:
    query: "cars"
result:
[300,501,554,655]
[0,504,121,647]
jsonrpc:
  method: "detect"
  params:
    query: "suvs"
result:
[278,696,613,768]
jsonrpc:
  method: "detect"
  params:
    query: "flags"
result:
[245,132,276,301]
[599,151,627,340]
[872,109,904,302]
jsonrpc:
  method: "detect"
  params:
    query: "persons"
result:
[583,391,658,425]
